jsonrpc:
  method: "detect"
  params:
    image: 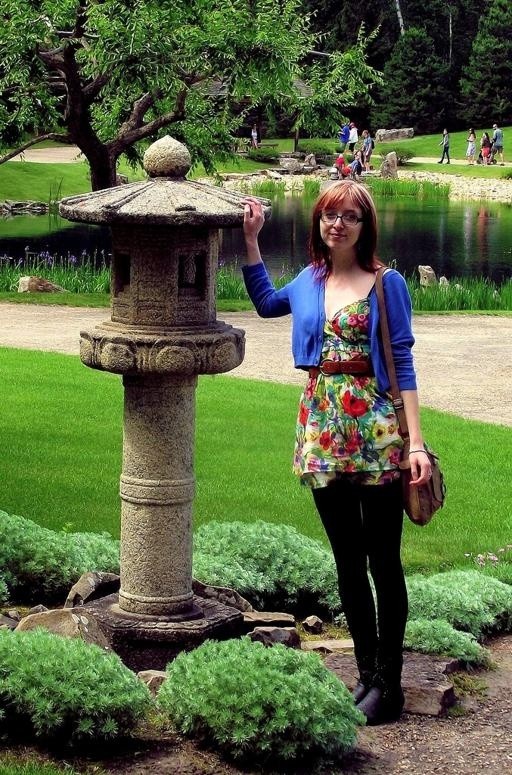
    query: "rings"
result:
[428,470,433,478]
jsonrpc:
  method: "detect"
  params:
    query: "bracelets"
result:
[407,449,430,456]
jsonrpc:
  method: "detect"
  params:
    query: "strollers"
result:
[476,140,497,164]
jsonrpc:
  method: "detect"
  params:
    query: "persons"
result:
[240,180,433,727]
[362,130,375,173]
[334,153,360,183]
[465,128,476,165]
[347,123,358,153]
[480,131,490,166]
[338,122,350,148]
[438,128,451,165]
[490,124,505,165]
[249,123,261,150]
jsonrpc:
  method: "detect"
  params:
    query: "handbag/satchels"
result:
[375,266,447,525]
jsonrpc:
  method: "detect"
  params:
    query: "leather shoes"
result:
[348,675,405,726]
[351,678,375,707]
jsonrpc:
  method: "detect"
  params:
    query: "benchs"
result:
[244,143,278,152]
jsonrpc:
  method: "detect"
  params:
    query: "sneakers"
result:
[445,162,450,164]
[437,161,443,164]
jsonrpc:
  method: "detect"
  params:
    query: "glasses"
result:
[320,212,365,228]
